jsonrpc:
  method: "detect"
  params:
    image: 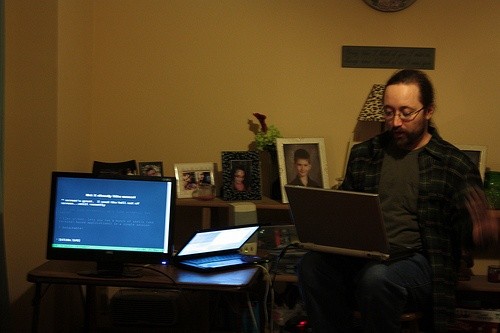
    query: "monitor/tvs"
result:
[454,144,487,185]
[46,172,176,278]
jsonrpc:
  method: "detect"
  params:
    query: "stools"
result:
[351,311,425,333]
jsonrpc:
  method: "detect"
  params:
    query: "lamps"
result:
[357,84,388,134]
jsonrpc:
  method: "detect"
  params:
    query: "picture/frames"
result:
[221,151,263,201]
[139,162,163,176]
[276,138,329,203]
[175,163,215,198]
[456,145,487,184]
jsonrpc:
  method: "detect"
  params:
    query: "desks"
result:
[26,259,272,333]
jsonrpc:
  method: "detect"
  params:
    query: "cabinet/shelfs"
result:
[92,195,500,333]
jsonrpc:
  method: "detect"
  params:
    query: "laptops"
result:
[284,185,413,262]
[173,223,267,274]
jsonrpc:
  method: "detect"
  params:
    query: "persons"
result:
[232,165,246,190]
[288,149,319,189]
[146,168,210,190]
[297,71,492,333]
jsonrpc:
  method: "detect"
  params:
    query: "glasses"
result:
[383,106,425,121]
